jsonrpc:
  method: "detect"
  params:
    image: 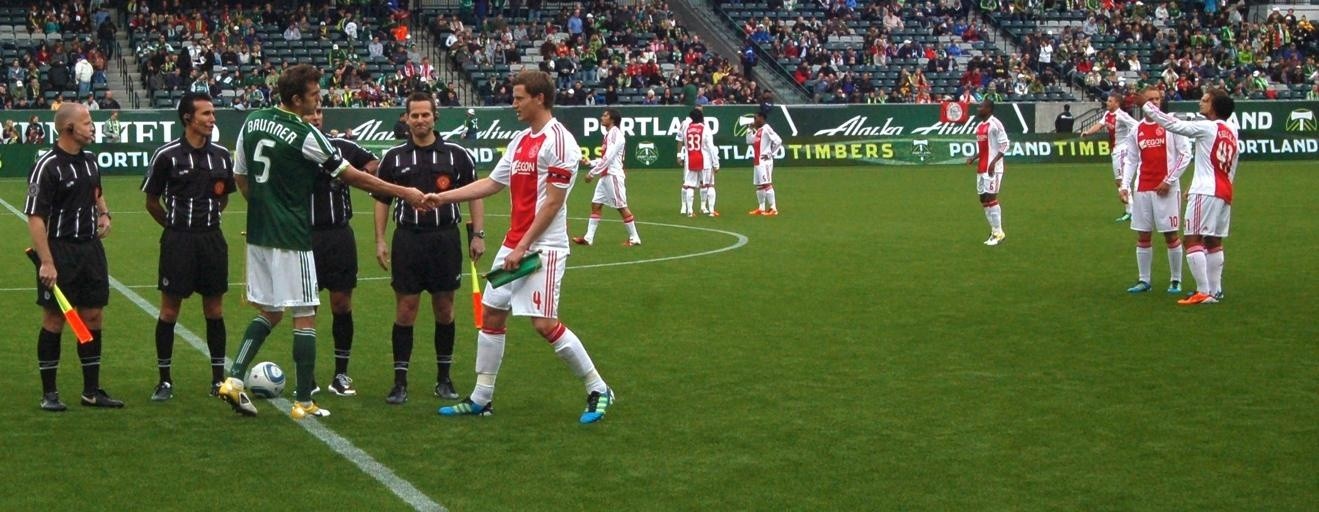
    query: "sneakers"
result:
[572,236,593,246]
[438,397,494,418]
[1166,278,1182,295]
[983,230,1007,248]
[150,382,175,401]
[1116,210,1133,224]
[433,380,461,400]
[622,240,642,248]
[80,387,125,409]
[762,208,779,216]
[680,209,696,218]
[1127,278,1154,293]
[580,386,616,425]
[292,382,323,397]
[1176,290,1225,305]
[209,375,258,420]
[328,374,356,399]
[748,207,766,216]
[386,386,410,407]
[700,208,719,217]
[290,399,332,420]
[39,390,67,411]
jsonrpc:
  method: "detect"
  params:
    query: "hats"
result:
[16,79,23,86]
[54,90,63,97]
[333,43,338,50]
[320,20,326,26]
[233,24,240,30]
[586,12,593,17]
[222,66,228,71]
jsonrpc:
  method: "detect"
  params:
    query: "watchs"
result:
[472,230,486,239]
[99,211,112,220]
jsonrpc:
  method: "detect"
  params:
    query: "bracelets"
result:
[361,167,369,172]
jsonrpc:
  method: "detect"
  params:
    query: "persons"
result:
[292,99,381,399]
[675,105,712,214]
[218,63,436,418]
[967,100,1010,246]
[1080,92,1140,222]
[684,109,721,218]
[373,89,487,407]
[572,108,643,246]
[1183,85,1240,295]
[1132,91,1240,305]
[412,69,616,423]
[747,112,782,216]
[25,102,128,410]
[138,93,238,402]
[1118,85,1192,294]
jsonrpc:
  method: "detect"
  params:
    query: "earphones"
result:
[434,115,439,121]
[67,126,73,133]
[185,117,192,122]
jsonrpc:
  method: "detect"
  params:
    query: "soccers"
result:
[248,361,285,398]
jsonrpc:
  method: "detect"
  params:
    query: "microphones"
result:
[428,121,437,131]
[73,130,92,140]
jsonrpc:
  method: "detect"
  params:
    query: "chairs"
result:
[21,1,1156,103]
